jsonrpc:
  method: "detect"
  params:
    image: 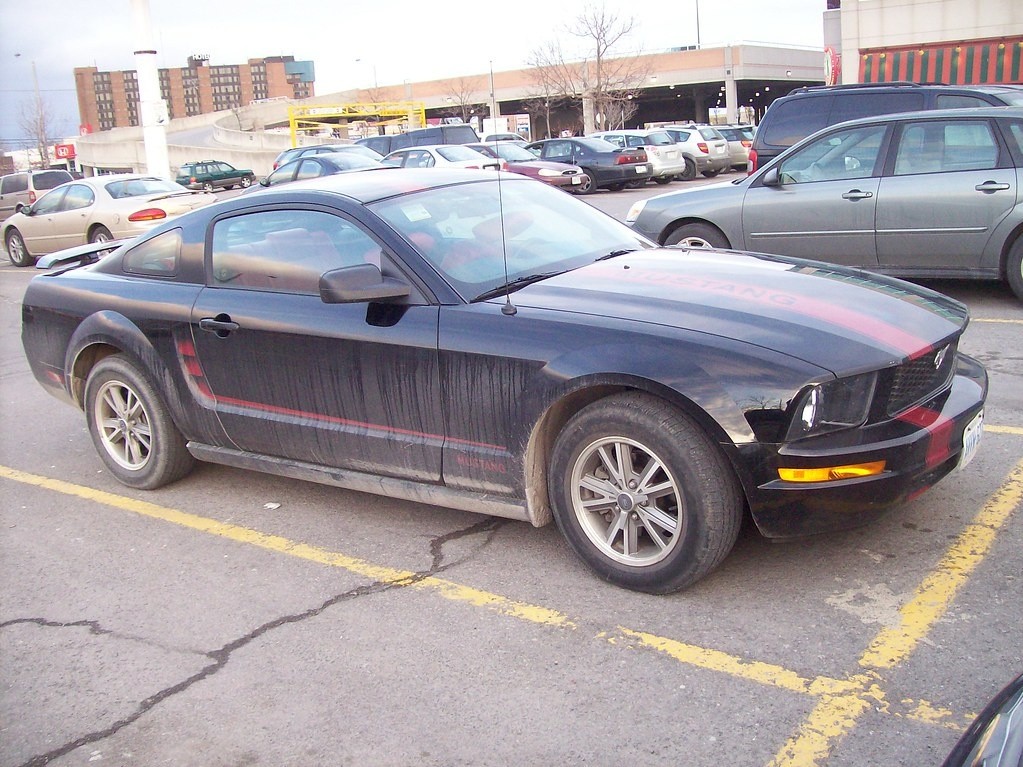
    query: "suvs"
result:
[480,132,529,144]
[175,160,255,194]
[587,129,686,184]
[0,169,75,225]
[353,125,479,156]
[746,82,1023,180]
[710,126,754,172]
[646,125,731,181]
[273,143,385,170]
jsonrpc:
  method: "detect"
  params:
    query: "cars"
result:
[0,174,218,268]
[379,144,509,173]
[20,166,988,594]
[241,153,401,196]
[623,107,1023,301]
[460,142,587,191]
[522,137,653,194]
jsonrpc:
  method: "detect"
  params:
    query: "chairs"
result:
[264,229,435,285]
